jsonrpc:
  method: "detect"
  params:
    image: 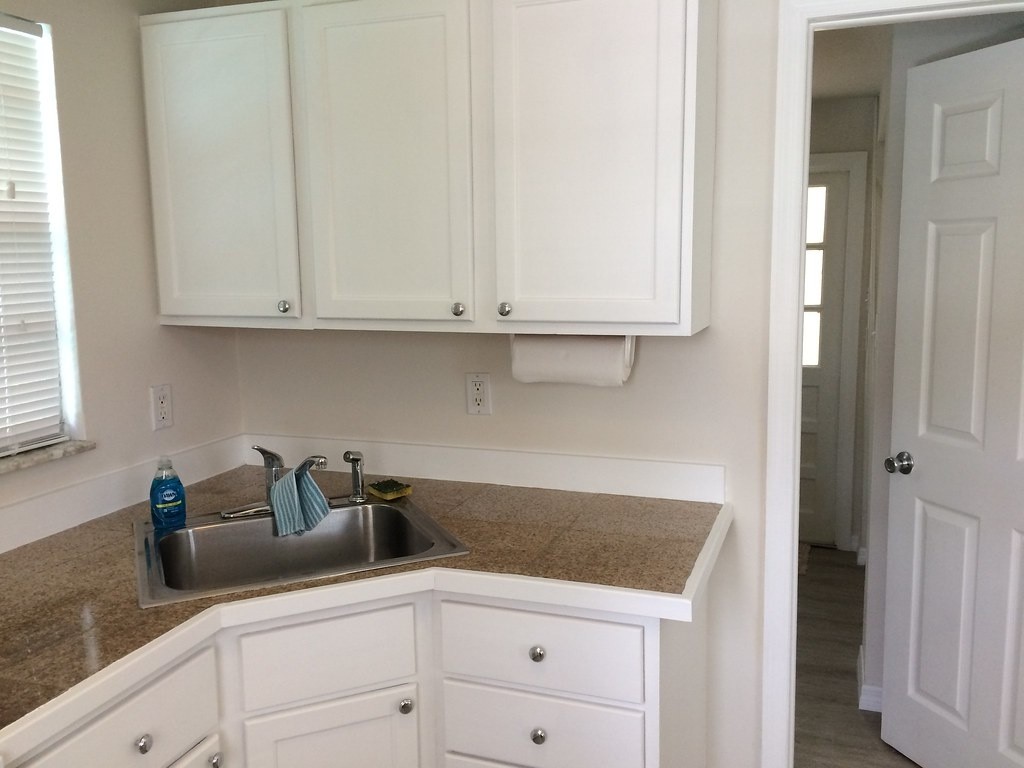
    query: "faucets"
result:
[252,445,326,503]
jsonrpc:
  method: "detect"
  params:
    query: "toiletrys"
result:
[148,453,186,530]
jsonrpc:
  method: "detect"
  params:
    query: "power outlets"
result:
[150,385,174,431]
[465,372,493,416]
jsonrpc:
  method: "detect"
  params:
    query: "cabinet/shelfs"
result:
[19,588,712,768]
[300,0,719,336]
[139,0,308,330]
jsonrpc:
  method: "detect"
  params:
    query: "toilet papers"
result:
[511,335,631,386]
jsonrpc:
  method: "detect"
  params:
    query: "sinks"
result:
[132,492,472,610]
